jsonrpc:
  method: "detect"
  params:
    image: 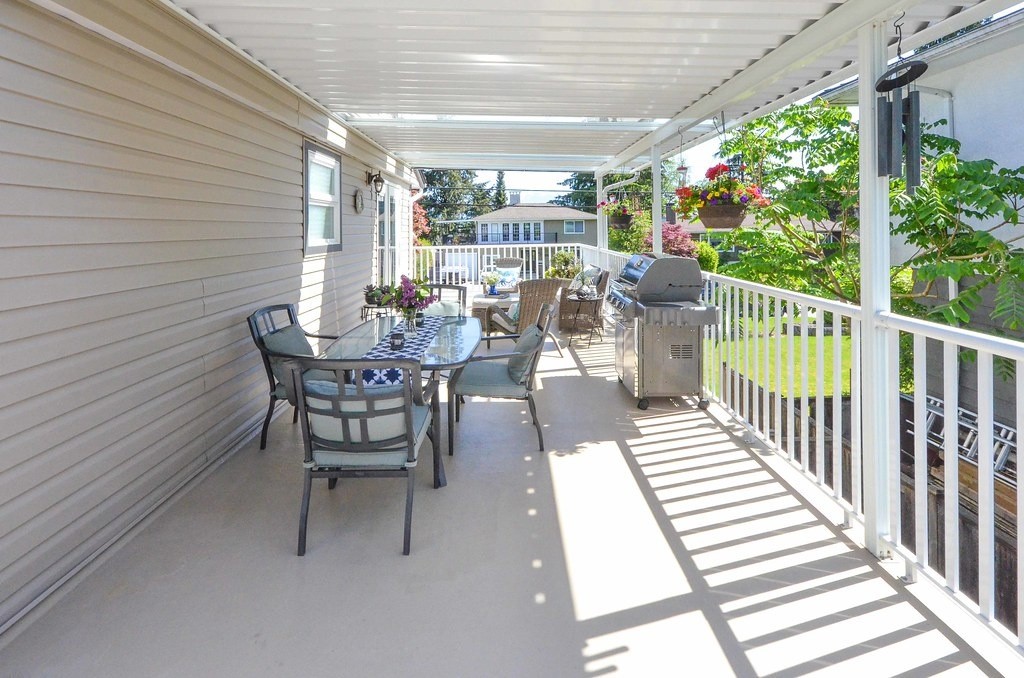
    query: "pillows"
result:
[263,323,315,385]
[303,379,418,452]
[507,323,543,385]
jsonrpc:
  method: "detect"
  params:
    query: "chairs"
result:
[422,283,468,313]
[283,356,447,555]
[483,257,523,296]
[485,278,565,359]
[247,304,340,449]
[447,303,556,455]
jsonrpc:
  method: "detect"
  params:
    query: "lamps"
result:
[366,168,384,193]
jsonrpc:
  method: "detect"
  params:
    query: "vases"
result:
[610,214,634,229]
[489,284,499,295]
[402,307,419,340]
[698,205,746,228]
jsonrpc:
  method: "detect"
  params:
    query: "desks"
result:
[567,292,604,349]
[364,304,388,322]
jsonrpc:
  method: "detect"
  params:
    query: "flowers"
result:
[671,162,772,223]
[484,271,502,286]
[596,194,643,216]
[374,274,439,318]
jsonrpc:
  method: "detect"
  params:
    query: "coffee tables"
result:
[472,293,520,332]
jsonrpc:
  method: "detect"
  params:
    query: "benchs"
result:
[555,264,610,334]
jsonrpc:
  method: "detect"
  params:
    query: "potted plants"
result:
[362,284,376,303]
[577,271,591,299]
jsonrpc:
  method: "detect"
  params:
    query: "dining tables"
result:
[313,314,481,456]
